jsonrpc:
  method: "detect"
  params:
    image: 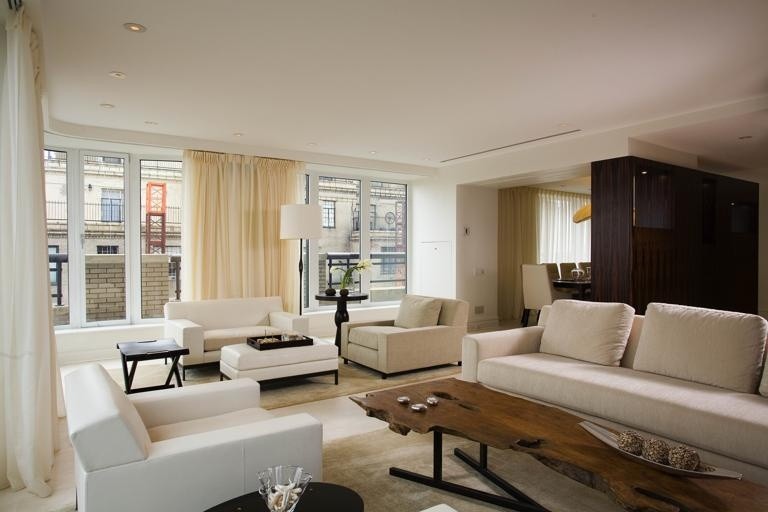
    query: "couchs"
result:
[459,300,768,486]
[164,298,310,380]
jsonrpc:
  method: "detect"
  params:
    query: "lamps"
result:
[280,203,324,313]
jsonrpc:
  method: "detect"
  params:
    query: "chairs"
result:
[59,361,323,512]
[340,294,468,379]
[519,262,592,328]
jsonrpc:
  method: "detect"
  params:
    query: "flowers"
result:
[330,260,375,289]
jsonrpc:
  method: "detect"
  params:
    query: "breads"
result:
[642,438,669,466]
[618,430,645,456]
[667,445,699,471]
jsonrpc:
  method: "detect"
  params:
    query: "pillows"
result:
[540,298,636,367]
[634,303,768,393]
[757,351,768,398]
[394,293,445,328]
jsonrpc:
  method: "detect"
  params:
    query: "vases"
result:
[342,277,355,290]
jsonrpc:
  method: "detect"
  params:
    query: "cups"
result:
[258,464,312,512]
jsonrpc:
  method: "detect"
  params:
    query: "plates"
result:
[579,420,743,482]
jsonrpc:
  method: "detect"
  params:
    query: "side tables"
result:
[115,338,189,395]
[315,292,368,357]
[204,481,365,511]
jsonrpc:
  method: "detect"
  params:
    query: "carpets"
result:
[322,425,637,512]
